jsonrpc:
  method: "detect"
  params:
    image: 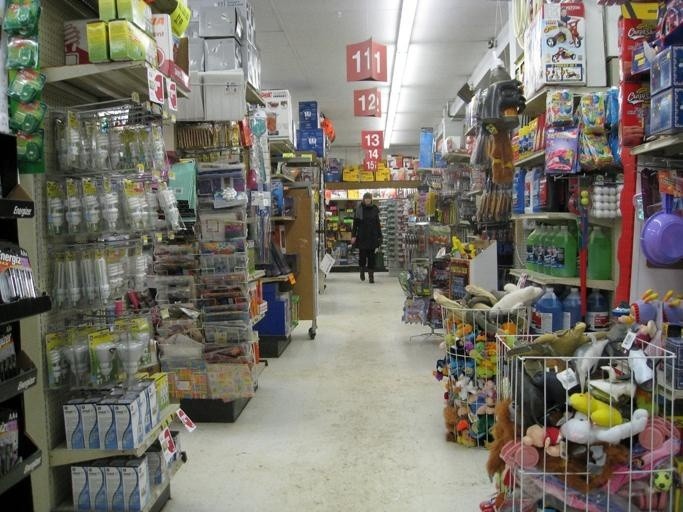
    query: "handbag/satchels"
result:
[375,251,384,272]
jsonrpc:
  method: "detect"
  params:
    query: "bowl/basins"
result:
[640,193,683,267]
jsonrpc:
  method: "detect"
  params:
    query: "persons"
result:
[350,192,384,283]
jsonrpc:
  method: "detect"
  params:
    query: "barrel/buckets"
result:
[544,225,560,275]
[562,287,583,330]
[533,223,547,272]
[524,220,542,272]
[534,287,563,334]
[584,288,609,332]
[529,286,548,331]
[585,225,612,280]
[551,226,577,277]
[537,223,552,273]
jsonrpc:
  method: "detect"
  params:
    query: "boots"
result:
[359,267,366,281]
[369,272,374,284]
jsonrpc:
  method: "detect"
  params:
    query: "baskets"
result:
[440,300,532,449]
[492,332,675,512]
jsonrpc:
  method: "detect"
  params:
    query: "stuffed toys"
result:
[430,280,683,512]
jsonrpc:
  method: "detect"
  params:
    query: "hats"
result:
[363,193,372,205]
